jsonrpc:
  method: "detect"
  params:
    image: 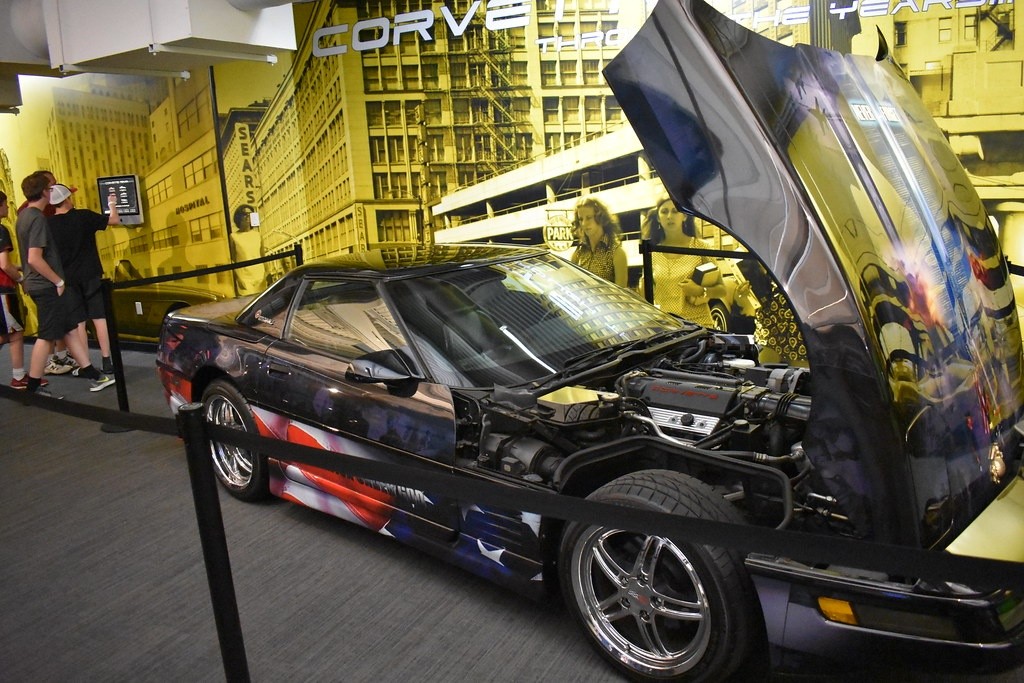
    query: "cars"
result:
[85,258,228,343]
[151,0,1024,682]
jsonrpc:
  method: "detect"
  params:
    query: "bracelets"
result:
[108,201,116,205]
[56,279,65,288]
[700,287,707,298]
[16,275,23,282]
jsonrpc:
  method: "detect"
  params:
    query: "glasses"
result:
[47,187,53,191]
[242,211,252,216]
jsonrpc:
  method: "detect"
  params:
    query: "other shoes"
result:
[105,363,114,374]
[72,367,92,378]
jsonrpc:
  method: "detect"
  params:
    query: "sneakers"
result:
[58,356,80,367]
[24,387,65,406]
[11,373,48,389]
[42,354,73,375]
[88,374,116,392]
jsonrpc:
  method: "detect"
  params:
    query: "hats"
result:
[49,185,72,205]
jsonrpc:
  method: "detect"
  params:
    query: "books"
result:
[686,262,719,306]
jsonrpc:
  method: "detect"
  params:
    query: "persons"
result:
[706,258,810,368]
[227,204,274,298]
[635,194,726,329]
[570,196,628,288]
[0,169,120,408]
